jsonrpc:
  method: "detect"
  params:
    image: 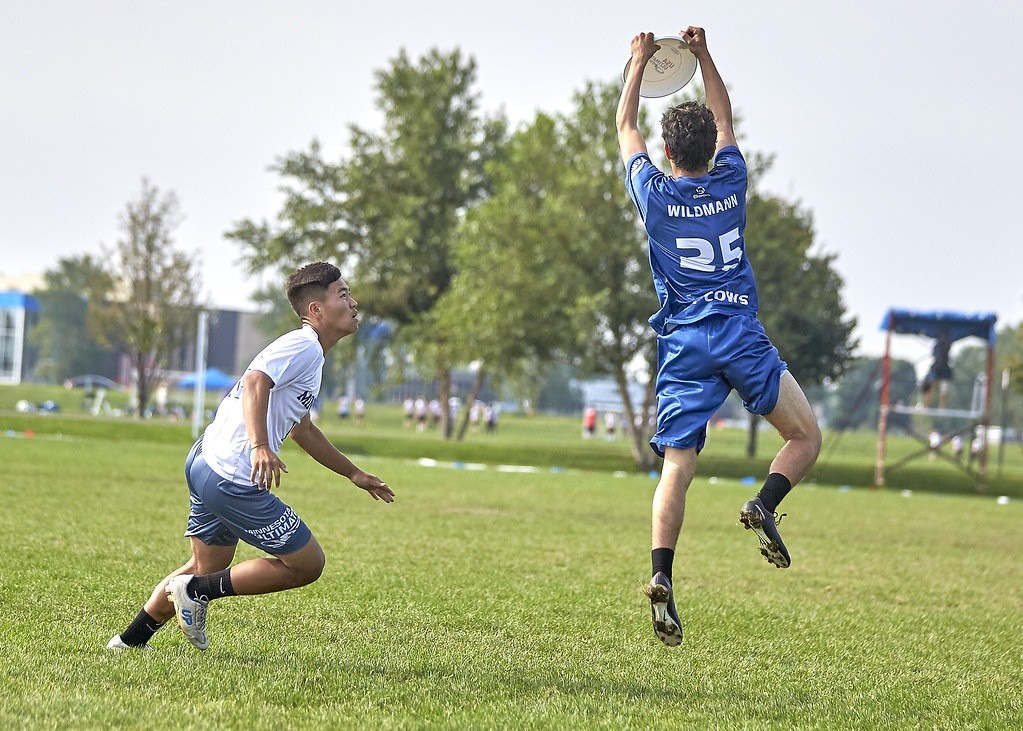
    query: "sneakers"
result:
[107,634,154,652]
[164,574,209,650]
[643,572,683,646]
[738,497,790,568]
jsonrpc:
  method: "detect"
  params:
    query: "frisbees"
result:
[621,36,698,99]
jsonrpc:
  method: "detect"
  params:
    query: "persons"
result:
[616,26,822,646]
[951,435,963,462]
[914,328,954,409]
[583,404,598,440]
[107,262,395,651]
[355,393,366,426]
[469,399,496,436]
[605,412,616,442]
[403,396,459,432]
[336,392,351,420]
[929,432,941,461]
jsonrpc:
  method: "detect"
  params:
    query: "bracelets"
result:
[251,443,269,450]
